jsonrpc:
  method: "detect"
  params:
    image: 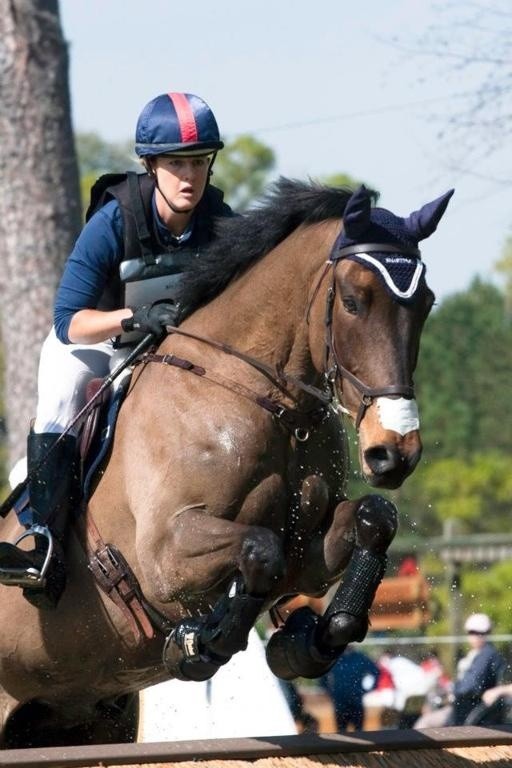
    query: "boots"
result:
[0,430,84,613]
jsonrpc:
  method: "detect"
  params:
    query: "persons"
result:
[376,651,426,731]
[0,92,246,610]
[440,618,512,729]
[277,674,317,736]
[418,650,457,713]
[318,646,379,732]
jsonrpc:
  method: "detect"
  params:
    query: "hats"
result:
[464,613,491,634]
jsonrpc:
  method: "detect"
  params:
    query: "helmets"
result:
[134,91,225,157]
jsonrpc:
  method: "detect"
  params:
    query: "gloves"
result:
[120,297,181,337]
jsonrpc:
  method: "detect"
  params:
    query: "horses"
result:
[0,173,456,750]
